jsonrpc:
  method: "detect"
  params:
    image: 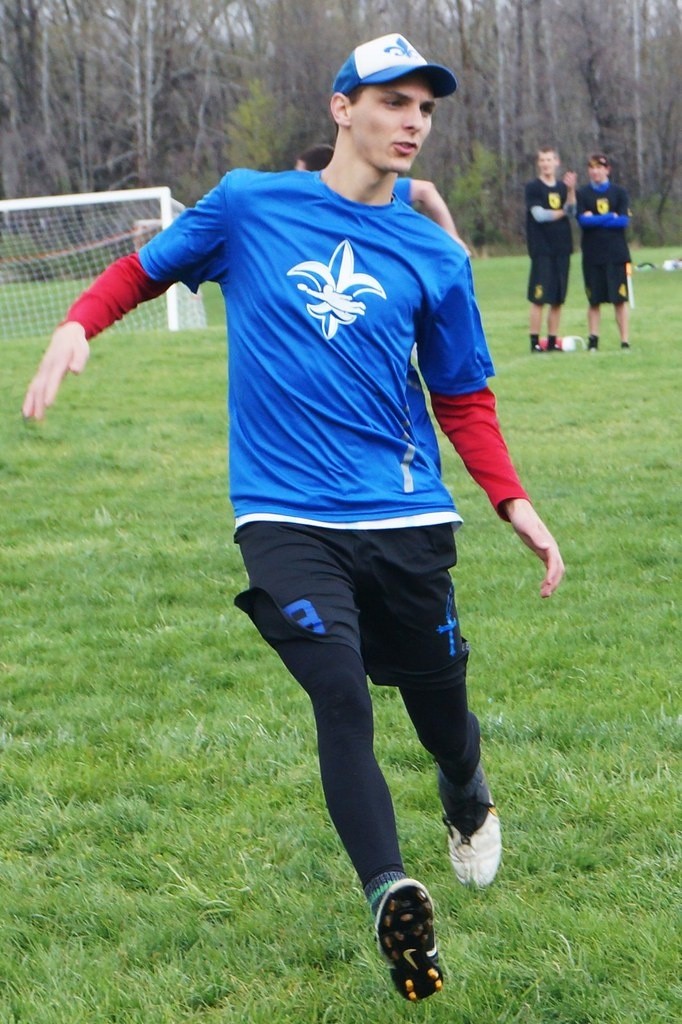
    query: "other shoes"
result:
[588,346,598,352]
[620,345,631,352]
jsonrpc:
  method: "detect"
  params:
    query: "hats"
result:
[332,33,457,98]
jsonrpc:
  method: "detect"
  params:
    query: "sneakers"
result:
[376,878,444,1003]
[442,789,502,888]
[531,343,561,352]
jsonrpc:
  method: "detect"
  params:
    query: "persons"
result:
[577,156,632,354]
[20,32,567,1004]
[524,146,578,354]
